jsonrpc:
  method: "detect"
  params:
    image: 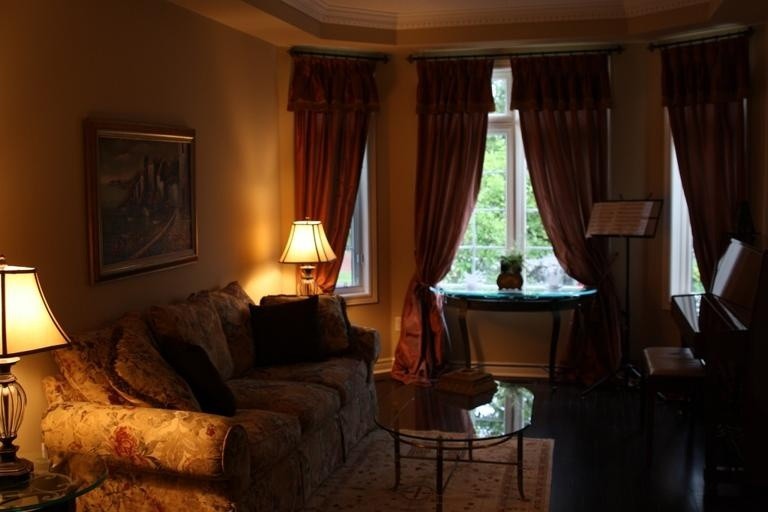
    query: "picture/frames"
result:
[81,122,200,289]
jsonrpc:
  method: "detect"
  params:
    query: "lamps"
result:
[277,216,340,298]
[1,251,75,486]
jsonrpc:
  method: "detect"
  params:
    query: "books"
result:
[437,380,496,394]
[440,368,492,384]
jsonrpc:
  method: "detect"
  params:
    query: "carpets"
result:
[297,425,557,512]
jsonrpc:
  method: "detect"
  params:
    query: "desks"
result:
[0,447,111,512]
[441,288,598,389]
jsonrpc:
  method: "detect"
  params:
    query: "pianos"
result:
[672,237,767,480]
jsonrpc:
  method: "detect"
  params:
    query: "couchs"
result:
[39,277,380,512]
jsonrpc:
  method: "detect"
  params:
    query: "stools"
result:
[638,345,704,462]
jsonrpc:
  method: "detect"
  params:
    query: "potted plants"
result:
[492,255,526,291]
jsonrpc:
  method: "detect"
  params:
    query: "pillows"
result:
[247,292,328,370]
[187,278,262,382]
[257,293,362,361]
[99,324,203,412]
[49,303,162,408]
[168,339,237,417]
[142,286,242,383]
